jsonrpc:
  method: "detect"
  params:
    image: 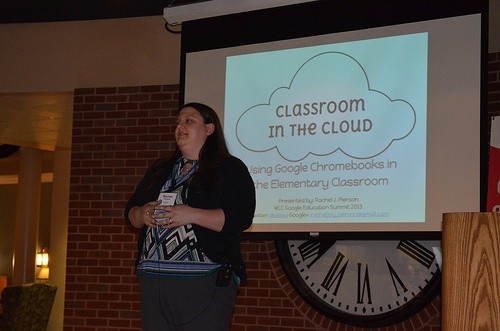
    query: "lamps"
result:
[36,248,48,267]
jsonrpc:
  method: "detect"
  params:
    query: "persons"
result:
[124,102,256,331]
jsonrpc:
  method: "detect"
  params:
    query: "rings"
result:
[169,218,172,224]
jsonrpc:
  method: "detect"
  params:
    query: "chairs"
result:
[0,283,57,331]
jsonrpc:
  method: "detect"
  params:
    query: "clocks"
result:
[274,239,443,328]
